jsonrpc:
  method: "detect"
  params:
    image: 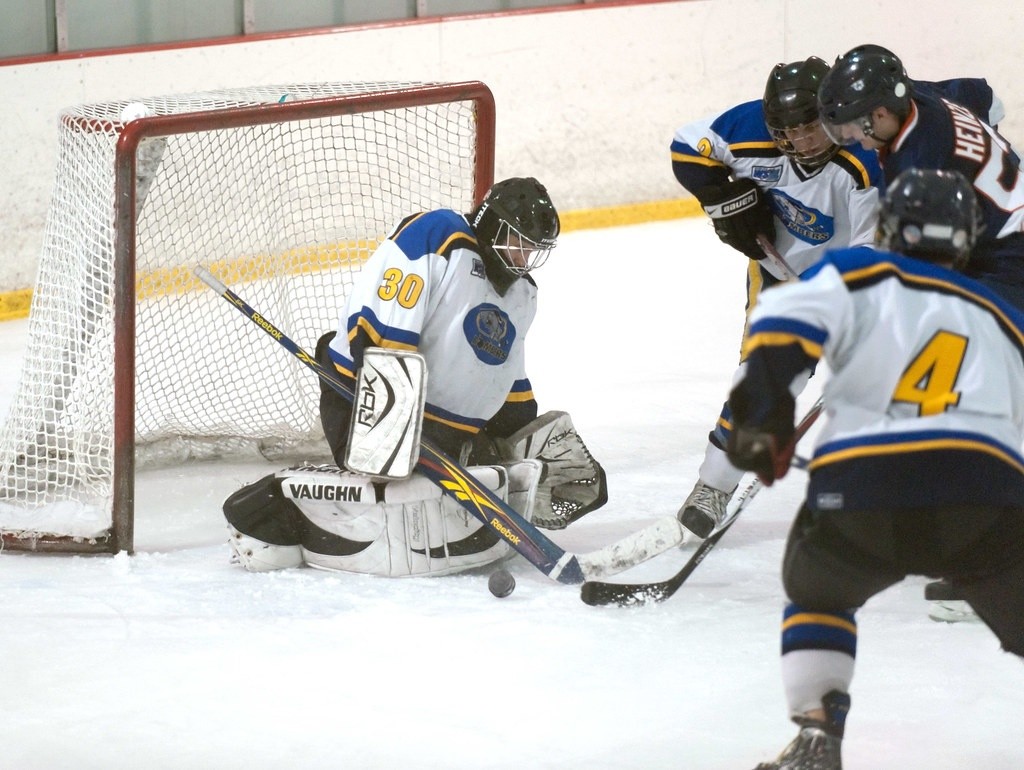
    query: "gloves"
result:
[701,177,776,260]
[728,354,795,487]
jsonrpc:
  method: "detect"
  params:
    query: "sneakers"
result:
[751,689,851,770]
[677,479,739,549]
[924,579,981,623]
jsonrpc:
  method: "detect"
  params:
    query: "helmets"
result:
[880,165,979,249]
[817,44,908,125]
[483,177,560,247]
[763,56,831,129]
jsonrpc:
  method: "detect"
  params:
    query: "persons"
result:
[223,176,609,580]
[667,43,1023,769]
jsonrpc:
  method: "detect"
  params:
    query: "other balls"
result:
[488,568,517,599]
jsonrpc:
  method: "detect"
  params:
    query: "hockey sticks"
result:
[705,431,814,471]
[190,259,685,586]
[576,395,827,607]
